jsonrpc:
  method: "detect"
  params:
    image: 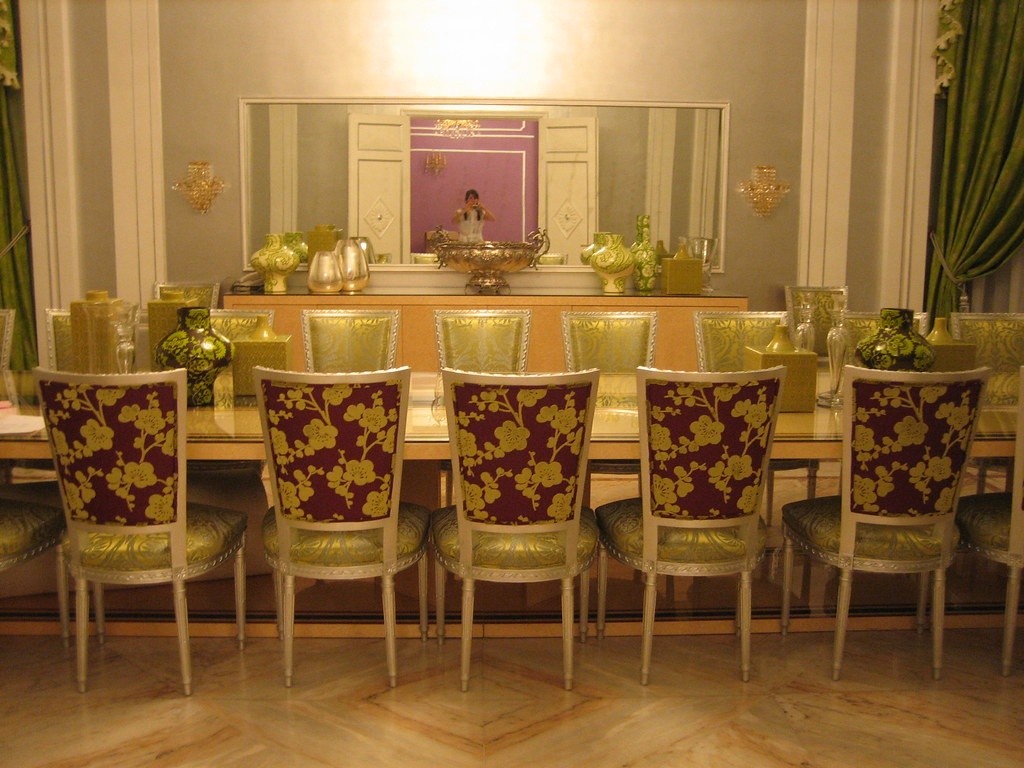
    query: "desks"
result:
[0,400,1024,642]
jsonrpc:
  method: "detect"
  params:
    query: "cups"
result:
[690,238,718,291]
[308,251,342,295]
[334,240,369,295]
[352,237,375,263]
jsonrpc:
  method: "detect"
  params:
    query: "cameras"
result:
[471,204,478,209]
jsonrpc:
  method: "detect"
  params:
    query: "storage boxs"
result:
[661,256,704,296]
[307,229,344,272]
[743,345,818,414]
[230,335,290,407]
[927,340,977,372]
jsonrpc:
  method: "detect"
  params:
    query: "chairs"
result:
[785,283,849,358]
[693,311,818,528]
[44,305,73,372]
[409,251,439,264]
[954,365,1024,679]
[301,307,401,375]
[432,307,534,581]
[153,280,220,309]
[0,309,17,400]
[535,252,569,265]
[562,310,676,611]
[373,253,394,263]
[950,311,1024,493]
[210,306,275,408]
[778,363,992,682]
[429,367,602,692]
[31,366,247,695]
[0,498,70,639]
[595,364,789,688]
[252,363,432,685]
[841,312,930,369]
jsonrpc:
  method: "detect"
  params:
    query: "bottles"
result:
[655,240,668,265]
[251,314,275,340]
[673,243,689,259]
[927,318,954,344]
[766,325,793,350]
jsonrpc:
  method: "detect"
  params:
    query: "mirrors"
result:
[237,97,729,273]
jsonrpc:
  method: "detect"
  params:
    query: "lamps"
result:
[171,161,226,216]
[737,165,791,220]
[435,118,482,139]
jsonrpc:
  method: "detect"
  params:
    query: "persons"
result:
[451,190,496,244]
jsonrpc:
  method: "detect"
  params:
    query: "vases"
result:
[250,233,300,295]
[580,231,611,265]
[590,234,635,293]
[766,326,798,353]
[854,307,937,371]
[631,215,643,252]
[923,317,954,343]
[631,215,659,291]
[151,306,232,408]
[283,231,309,263]
